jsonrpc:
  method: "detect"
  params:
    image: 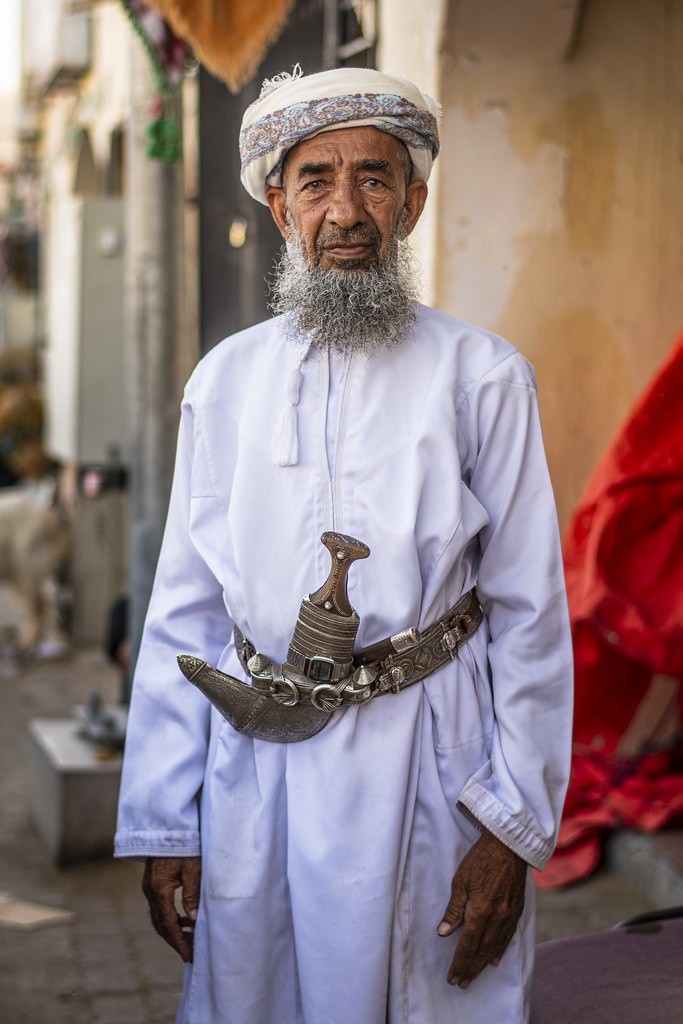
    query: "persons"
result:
[115,62,573,1024]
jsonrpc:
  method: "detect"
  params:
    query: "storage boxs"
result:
[26,717,124,854]
